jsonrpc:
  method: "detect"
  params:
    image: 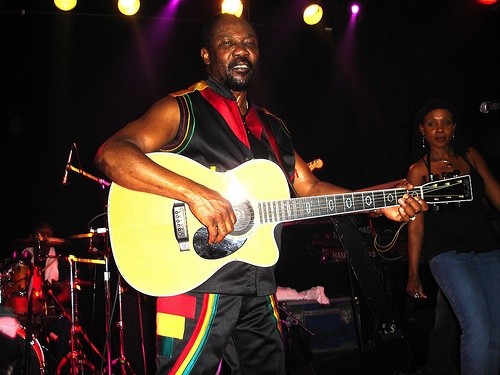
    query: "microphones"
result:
[479,101,500,114]
[60,144,73,186]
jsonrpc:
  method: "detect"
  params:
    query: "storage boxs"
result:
[279,297,359,353]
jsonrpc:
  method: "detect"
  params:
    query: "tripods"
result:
[54,235,139,375]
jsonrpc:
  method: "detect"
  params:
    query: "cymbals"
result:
[17,236,64,245]
[70,233,108,239]
[58,281,94,284]
[47,255,106,265]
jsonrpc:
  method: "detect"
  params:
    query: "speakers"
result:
[275,297,364,375]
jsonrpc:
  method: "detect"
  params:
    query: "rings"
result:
[410,215,416,220]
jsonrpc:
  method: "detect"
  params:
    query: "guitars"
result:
[106,150,475,298]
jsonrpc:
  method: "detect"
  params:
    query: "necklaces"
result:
[435,151,453,168]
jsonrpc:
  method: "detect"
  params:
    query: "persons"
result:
[408,101,500,375]
[18,224,61,302]
[94,14,429,375]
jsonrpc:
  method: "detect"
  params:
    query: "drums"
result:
[2,265,48,317]
[48,284,71,307]
[0,316,45,375]
